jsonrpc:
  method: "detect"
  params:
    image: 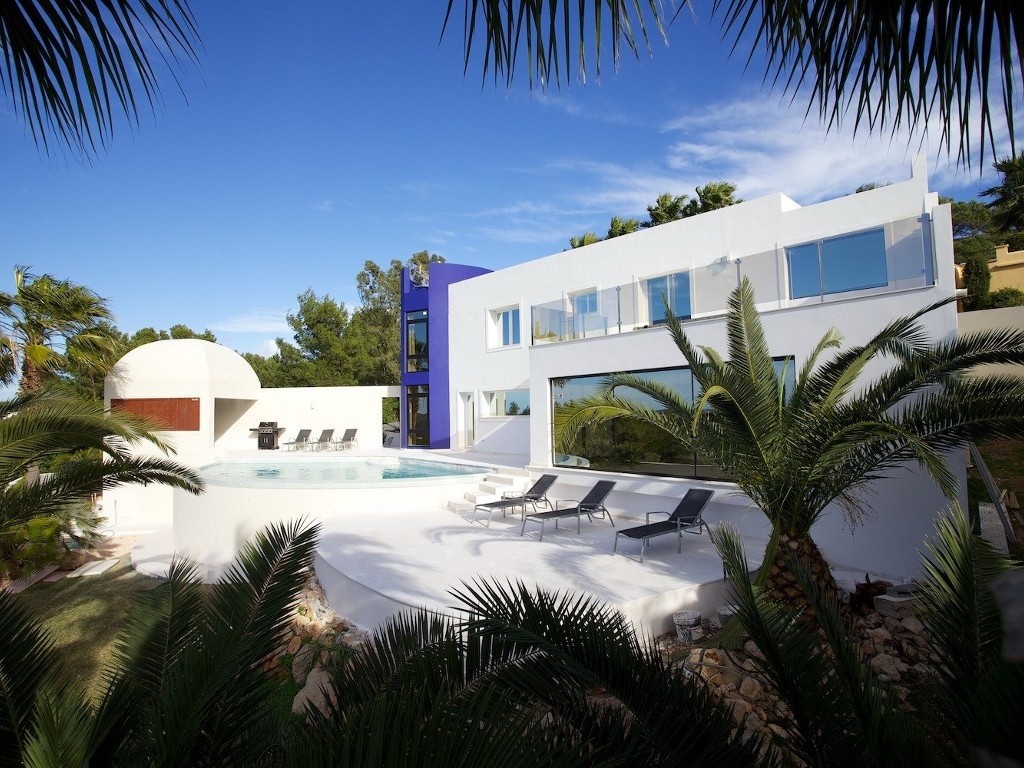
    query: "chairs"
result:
[333,429,358,451]
[472,474,559,528]
[613,489,716,563]
[308,430,334,452]
[521,480,618,542]
[282,430,311,452]
